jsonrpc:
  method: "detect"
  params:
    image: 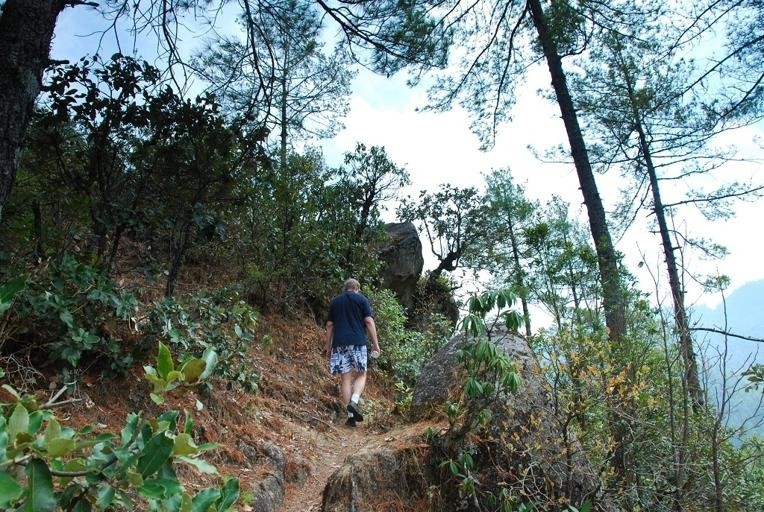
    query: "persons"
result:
[324,278,382,428]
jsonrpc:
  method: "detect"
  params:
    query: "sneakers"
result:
[347,401,363,421]
[345,417,355,426]
